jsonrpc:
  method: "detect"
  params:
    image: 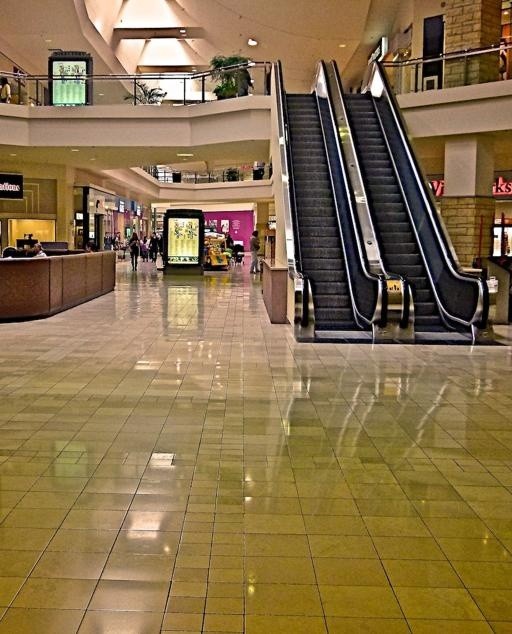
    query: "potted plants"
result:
[209,54,253,100]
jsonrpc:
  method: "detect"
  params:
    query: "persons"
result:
[252,161,265,180]
[33,243,48,257]
[104,229,163,263]
[129,232,140,271]
[227,232,233,247]
[267,65,270,95]
[237,67,254,97]
[0,77,11,104]
[86,242,98,253]
[250,231,261,274]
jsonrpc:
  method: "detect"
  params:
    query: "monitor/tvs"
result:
[0,172,24,201]
